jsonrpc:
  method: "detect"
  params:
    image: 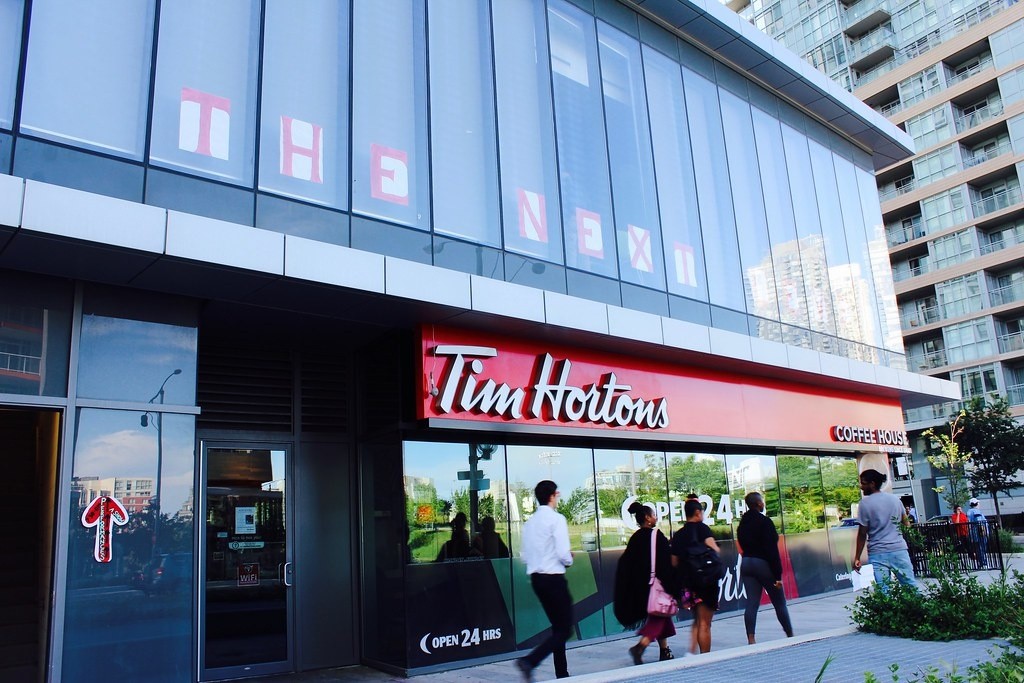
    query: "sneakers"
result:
[629,643,644,666]
[659,647,674,661]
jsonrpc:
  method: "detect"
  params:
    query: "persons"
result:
[736,492,794,644]
[952,505,976,560]
[448,512,509,559]
[613,502,677,666]
[854,469,921,597]
[905,505,916,524]
[967,498,989,567]
[515,480,575,683]
[671,493,726,656]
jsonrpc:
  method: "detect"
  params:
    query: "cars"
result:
[138,551,191,595]
[922,514,999,537]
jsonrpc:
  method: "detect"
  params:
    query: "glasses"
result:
[555,490,561,495]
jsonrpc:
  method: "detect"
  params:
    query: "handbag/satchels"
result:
[687,551,725,588]
[647,577,679,618]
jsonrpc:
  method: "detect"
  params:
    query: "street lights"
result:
[140,368,184,601]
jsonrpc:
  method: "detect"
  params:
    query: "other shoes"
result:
[981,565,992,569]
[516,658,536,683]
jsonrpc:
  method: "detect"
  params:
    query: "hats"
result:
[970,498,980,504]
